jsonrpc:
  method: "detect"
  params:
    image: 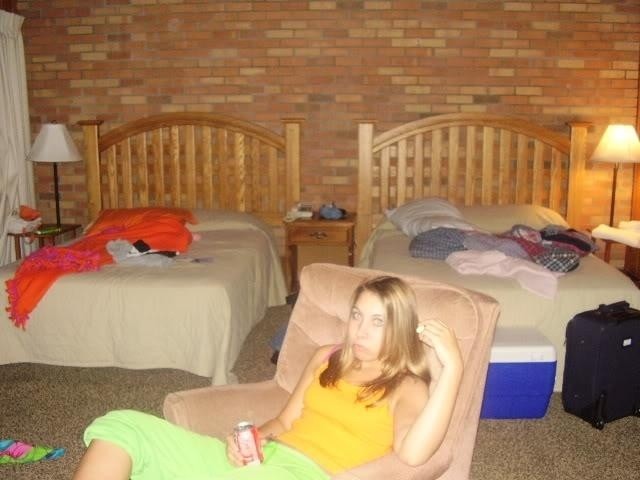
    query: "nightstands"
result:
[283,210,357,292]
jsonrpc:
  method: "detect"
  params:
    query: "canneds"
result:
[233,420,264,468]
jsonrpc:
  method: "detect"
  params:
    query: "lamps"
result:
[25,123,83,231]
[587,123,640,227]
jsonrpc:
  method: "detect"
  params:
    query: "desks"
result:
[592,238,628,271]
[7,224,80,260]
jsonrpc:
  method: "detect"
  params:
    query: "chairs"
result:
[163,261,500,480]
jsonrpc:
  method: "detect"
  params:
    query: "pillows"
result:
[84,208,195,237]
[384,197,476,238]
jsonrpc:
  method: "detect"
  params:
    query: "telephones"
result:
[282,202,314,223]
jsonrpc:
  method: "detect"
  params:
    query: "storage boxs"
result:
[479,326,557,420]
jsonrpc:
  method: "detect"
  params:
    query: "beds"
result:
[353,114,640,391]
[0,110,305,388]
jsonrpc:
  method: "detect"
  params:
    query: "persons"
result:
[74,275,464,480]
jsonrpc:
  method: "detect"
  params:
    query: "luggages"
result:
[561,300,640,431]
